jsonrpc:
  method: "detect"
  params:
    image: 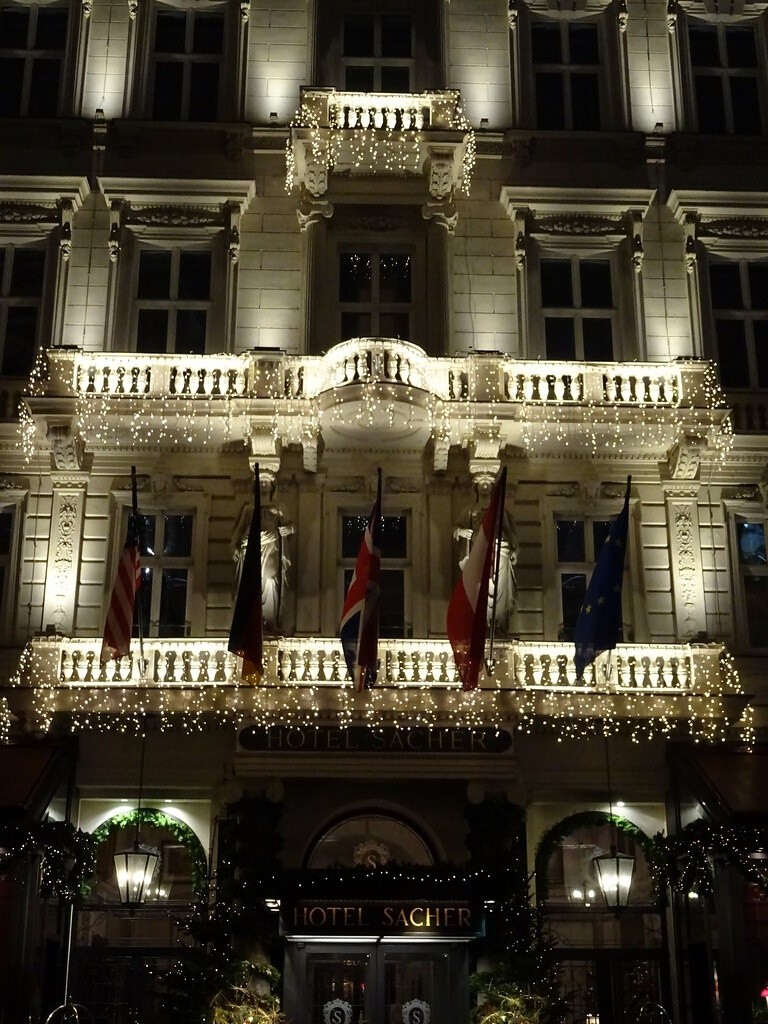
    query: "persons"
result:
[231,469,298,632]
[449,471,520,639]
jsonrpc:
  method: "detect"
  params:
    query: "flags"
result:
[100,511,142,669]
[574,505,629,683]
[446,477,503,693]
[340,500,382,694]
[227,486,265,685]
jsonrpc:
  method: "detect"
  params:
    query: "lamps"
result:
[112,713,160,918]
[697,631,707,642]
[95,109,105,120]
[270,111,279,122]
[654,122,664,134]
[593,734,636,918]
[480,118,488,130]
[46,624,55,635]
